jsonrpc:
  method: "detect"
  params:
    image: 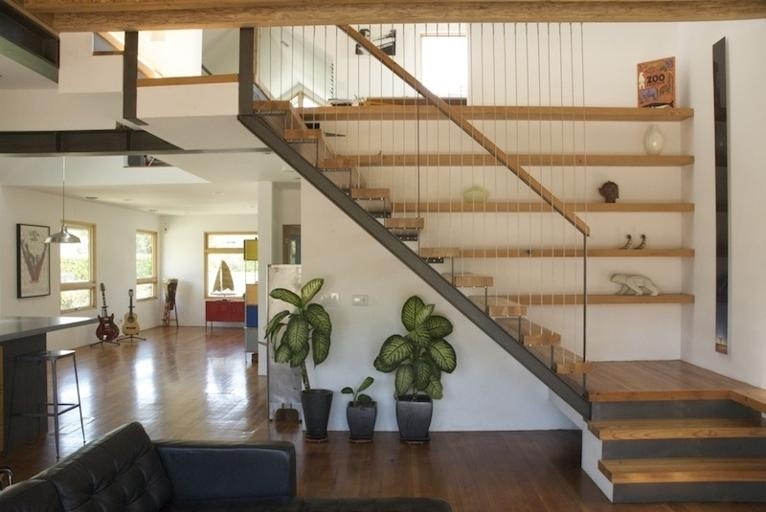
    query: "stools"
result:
[5,350,86,459]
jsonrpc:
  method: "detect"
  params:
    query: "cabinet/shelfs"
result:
[293,106,696,306]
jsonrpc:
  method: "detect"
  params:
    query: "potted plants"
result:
[261,277,458,446]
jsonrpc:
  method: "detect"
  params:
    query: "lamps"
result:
[44,154,80,244]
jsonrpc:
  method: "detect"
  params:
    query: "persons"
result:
[356,29,370,54]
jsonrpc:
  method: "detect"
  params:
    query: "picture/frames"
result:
[16,223,52,299]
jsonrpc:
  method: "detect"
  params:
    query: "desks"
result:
[205,299,245,335]
[1,316,100,449]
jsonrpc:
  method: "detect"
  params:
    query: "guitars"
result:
[122,289,140,336]
[96,283,119,342]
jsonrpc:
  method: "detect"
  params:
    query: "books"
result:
[637,56,676,108]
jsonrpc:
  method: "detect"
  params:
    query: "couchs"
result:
[0,420,451,511]
